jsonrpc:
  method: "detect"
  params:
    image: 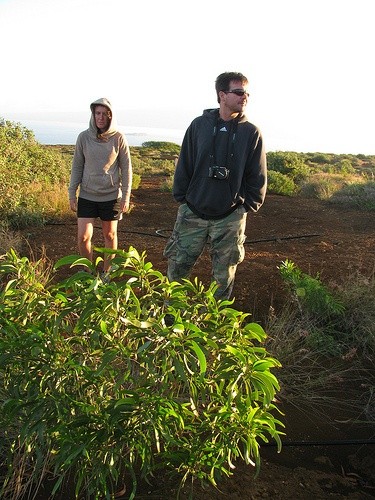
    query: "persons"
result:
[68,97,132,276]
[162,71,267,311]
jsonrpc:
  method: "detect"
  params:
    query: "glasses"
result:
[224,88,249,97]
